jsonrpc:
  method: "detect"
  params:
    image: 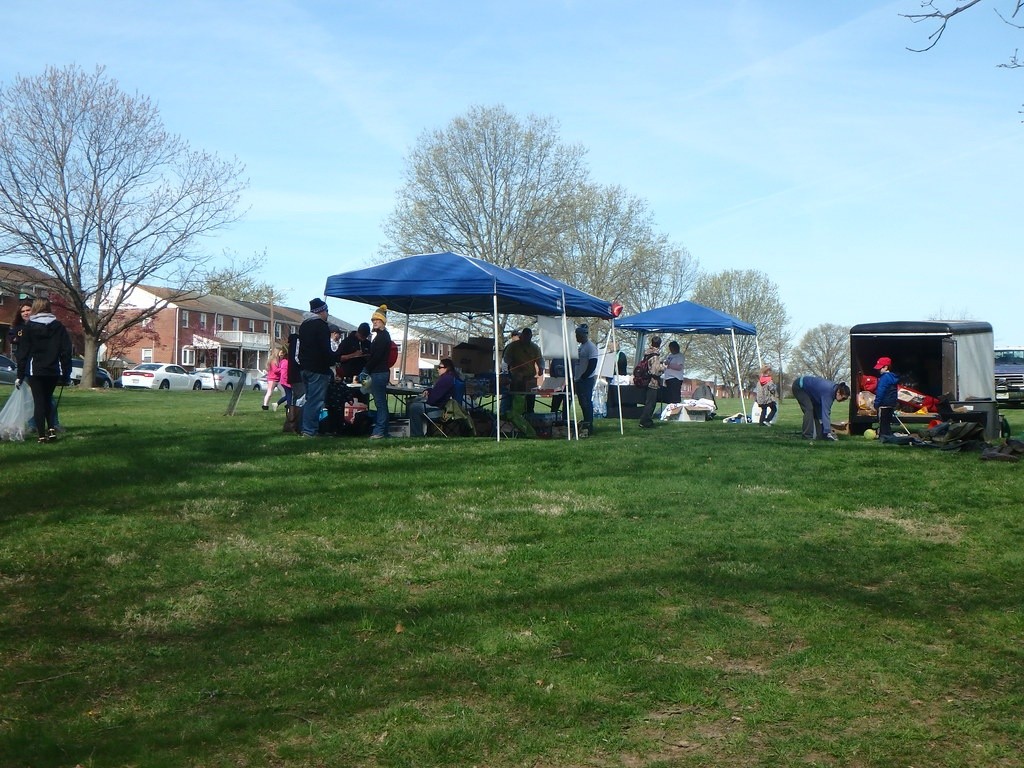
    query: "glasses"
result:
[439,366,447,369]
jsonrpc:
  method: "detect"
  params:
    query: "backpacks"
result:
[634,353,659,388]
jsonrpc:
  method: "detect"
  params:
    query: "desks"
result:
[491,392,571,423]
[348,383,425,416]
[949,401,997,441]
[608,385,670,419]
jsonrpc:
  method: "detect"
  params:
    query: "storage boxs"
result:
[831,420,849,435]
[319,402,368,424]
[532,412,574,437]
[453,337,495,373]
[476,373,512,393]
[531,377,565,392]
[667,406,711,422]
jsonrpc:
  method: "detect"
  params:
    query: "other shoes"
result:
[48,428,55,438]
[54,424,66,433]
[26,426,38,436]
[763,421,772,427]
[261,404,267,411]
[272,402,279,412]
[370,435,385,439]
[37,436,46,442]
[639,423,648,428]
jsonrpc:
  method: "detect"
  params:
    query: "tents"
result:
[324,252,624,441]
[614,301,756,424]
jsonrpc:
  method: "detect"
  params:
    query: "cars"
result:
[0,354,18,385]
[114,363,203,390]
[193,366,279,391]
[70,353,115,389]
[994,345,1024,404]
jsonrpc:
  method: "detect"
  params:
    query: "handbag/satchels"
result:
[389,340,398,368]
[283,405,301,433]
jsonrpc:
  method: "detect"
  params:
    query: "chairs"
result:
[490,395,538,439]
[424,375,487,436]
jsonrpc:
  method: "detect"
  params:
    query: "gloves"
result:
[62,374,72,386]
[826,432,835,441]
[15,378,22,390]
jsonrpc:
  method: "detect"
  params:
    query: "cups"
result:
[407,383,413,389]
[402,381,406,387]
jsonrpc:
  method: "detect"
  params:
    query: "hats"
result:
[310,298,328,314]
[371,304,387,324]
[511,331,522,337]
[575,323,590,334]
[329,323,344,333]
[358,323,371,335]
[874,357,891,370]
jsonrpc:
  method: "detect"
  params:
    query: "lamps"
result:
[198,356,204,362]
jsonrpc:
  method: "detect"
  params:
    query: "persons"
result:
[606,340,627,385]
[359,304,391,439]
[573,324,598,427]
[327,324,342,435]
[299,298,334,437]
[261,342,292,412]
[639,336,667,429]
[664,341,685,403]
[406,358,455,437]
[756,365,778,427]
[550,359,576,421]
[335,322,370,409]
[502,327,545,412]
[8,297,72,442]
[501,329,521,415]
[792,376,850,441]
[324,367,354,434]
[874,358,899,441]
